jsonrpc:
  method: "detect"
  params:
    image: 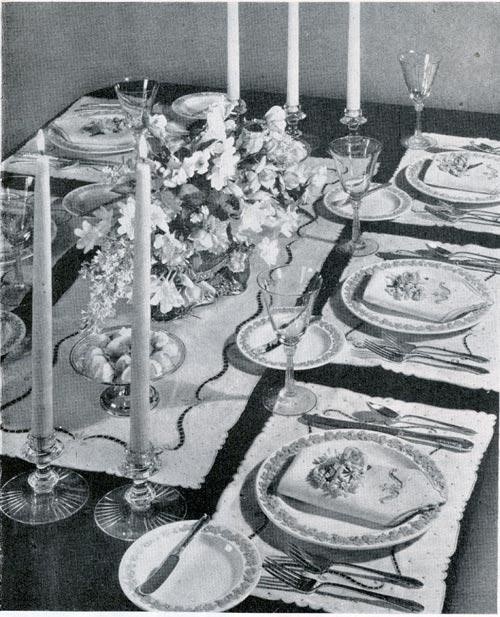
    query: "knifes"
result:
[301,411,473,454]
[332,180,393,208]
[246,314,322,358]
[134,512,212,598]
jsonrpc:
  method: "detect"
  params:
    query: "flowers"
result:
[73,103,330,342]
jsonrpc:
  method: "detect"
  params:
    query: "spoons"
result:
[366,401,479,437]
[470,140,500,153]
[424,243,500,265]
[262,541,429,613]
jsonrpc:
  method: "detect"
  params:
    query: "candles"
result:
[225,1,363,110]
[29,155,153,454]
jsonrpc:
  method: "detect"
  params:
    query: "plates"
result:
[171,91,247,121]
[45,109,137,157]
[340,258,493,336]
[61,182,133,221]
[403,150,500,207]
[0,310,27,358]
[320,181,414,221]
[234,311,346,373]
[115,517,262,613]
[254,427,448,553]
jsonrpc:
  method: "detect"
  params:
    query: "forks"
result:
[423,198,500,228]
[363,329,492,375]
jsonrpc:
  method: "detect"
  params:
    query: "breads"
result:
[82,326,176,386]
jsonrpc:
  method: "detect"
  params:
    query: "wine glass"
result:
[327,135,384,257]
[67,322,188,419]
[253,262,324,418]
[0,174,36,308]
[396,50,442,150]
[113,77,160,171]
